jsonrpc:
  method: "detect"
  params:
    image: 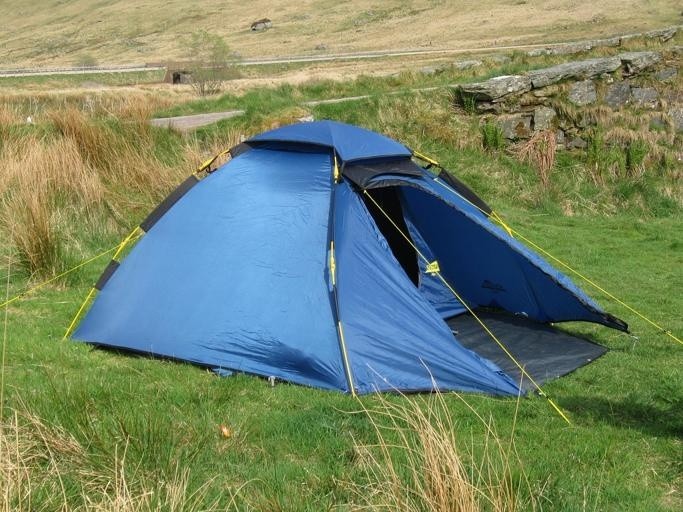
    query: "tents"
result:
[69,121,631,399]
